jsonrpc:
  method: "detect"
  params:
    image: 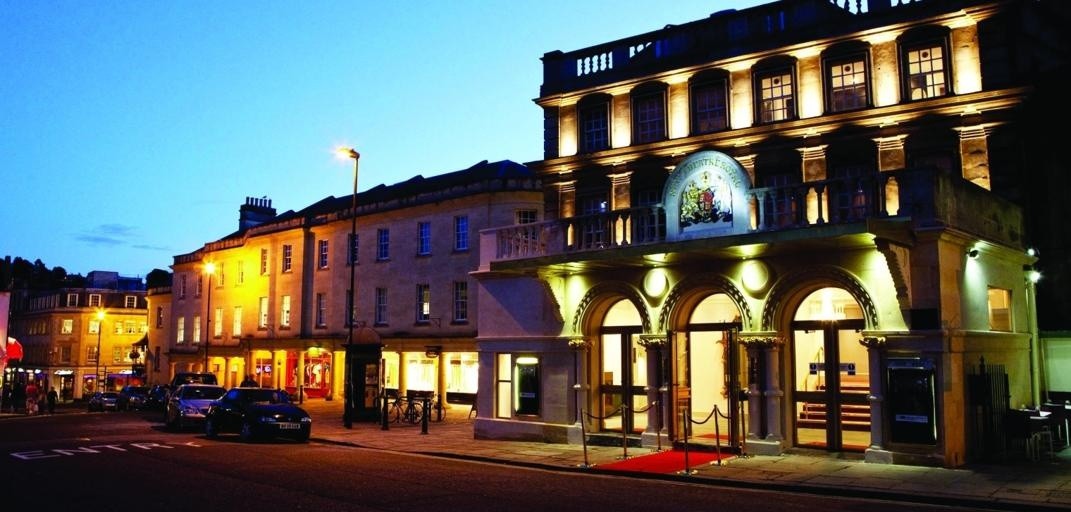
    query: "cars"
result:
[88,372,312,441]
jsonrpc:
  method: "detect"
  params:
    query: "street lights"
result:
[339,147,360,429]
[95,311,105,391]
[204,263,215,372]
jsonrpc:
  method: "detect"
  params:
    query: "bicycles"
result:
[381,391,446,425]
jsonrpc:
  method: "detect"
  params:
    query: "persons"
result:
[240,375,255,387]
[248,374,258,387]
[47,386,58,415]
[62,386,69,404]
[36,387,47,415]
